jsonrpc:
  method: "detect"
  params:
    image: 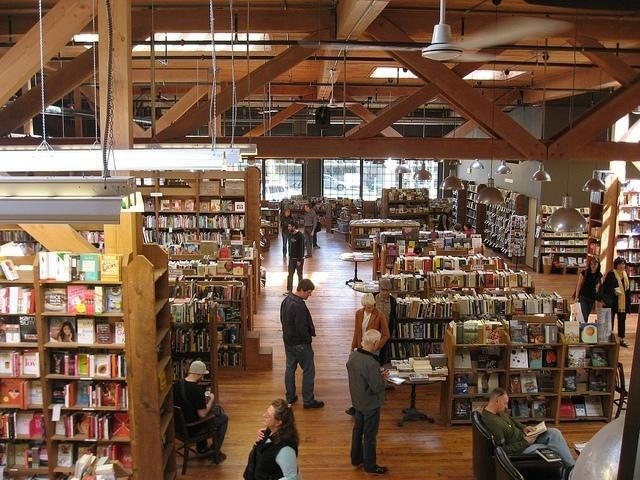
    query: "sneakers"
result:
[619,339,630,348]
[289,395,298,405]
[351,462,364,469]
[364,464,388,475]
[282,291,292,297]
[302,400,325,409]
[344,407,355,417]
[207,452,227,463]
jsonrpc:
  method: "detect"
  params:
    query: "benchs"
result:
[614,363,630,418]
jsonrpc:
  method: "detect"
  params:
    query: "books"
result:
[1,229,134,479]
[451,179,529,258]
[350,187,450,250]
[282,194,363,232]
[143,197,280,380]
[538,178,640,305]
[374,230,618,423]
[525,420,548,439]
[535,447,562,463]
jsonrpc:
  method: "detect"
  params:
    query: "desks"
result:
[340,252,372,286]
[351,281,378,296]
[385,363,440,427]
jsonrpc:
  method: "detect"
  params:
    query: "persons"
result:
[571,253,601,322]
[481,388,577,472]
[242,398,301,480]
[345,293,391,416]
[343,327,390,475]
[437,207,474,239]
[279,203,321,296]
[278,279,326,409]
[370,278,397,366]
[172,360,228,463]
[601,257,630,347]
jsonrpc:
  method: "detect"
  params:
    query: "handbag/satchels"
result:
[573,290,576,299]
[569,303,587,323]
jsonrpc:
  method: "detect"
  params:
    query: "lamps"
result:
[582,68,606,190]
[246,0,255,166]
[545,1,589,233]
[475,0,503,204]
[495,64,513,174]
[450,107,461,166]
[395,125,411,173]
[294,96,308,164]
[440,108,465,190]
[471,81,483,170]
[414,105,436,180]
[531,38,551,182]
[373,90,384,164]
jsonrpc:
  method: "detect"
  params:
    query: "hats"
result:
[186,360,210,375]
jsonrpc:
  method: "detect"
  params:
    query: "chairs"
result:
[174,408,218,475]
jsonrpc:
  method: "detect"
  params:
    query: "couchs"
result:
[495,447,565,480]
[471,411,542,480]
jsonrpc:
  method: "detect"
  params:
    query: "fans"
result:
[302,0,570,62]
[296,70,356,109]
